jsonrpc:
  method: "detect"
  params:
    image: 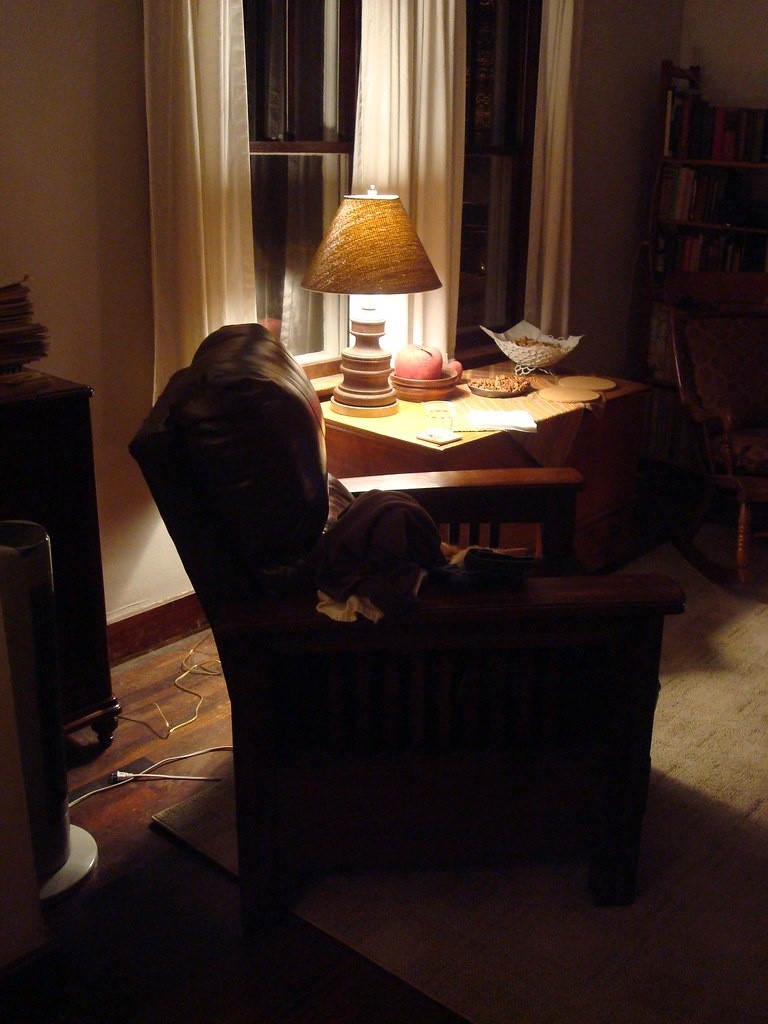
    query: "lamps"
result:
[301,186,443,418]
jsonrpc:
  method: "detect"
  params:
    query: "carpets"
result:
[150,530,767,1024]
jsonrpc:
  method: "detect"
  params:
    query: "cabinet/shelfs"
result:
[319,360,650,572]
[622,90,768,535]
[0,366,123,753]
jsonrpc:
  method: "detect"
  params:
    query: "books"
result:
[663,91,768,164]
[657,228,768,273]
[641,306,689,453]
[0,276,48,367]
[662,166,768,224]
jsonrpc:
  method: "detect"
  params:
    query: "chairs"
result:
[126,324,687,950]
[669,302,768,590]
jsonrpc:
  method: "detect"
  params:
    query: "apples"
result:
[394,344,442,380]
[442,360,463,385]
